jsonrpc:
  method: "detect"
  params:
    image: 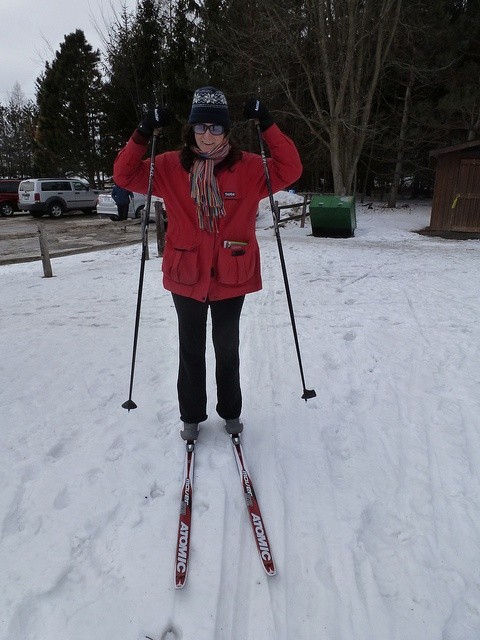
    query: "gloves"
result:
[137,106,175,136]
[242,96,275,132]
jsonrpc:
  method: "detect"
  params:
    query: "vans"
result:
[18,178,108,217]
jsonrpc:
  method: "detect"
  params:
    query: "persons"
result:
[112,184,133,220]
[112,85,304,441]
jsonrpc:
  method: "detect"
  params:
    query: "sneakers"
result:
[224,417,244,434]
[180,420,200,441]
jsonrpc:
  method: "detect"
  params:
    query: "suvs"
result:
[0,178,23,217]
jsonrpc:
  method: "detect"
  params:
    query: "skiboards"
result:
[174,438,277,590]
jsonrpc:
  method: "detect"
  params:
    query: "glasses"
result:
[192,123,225,135]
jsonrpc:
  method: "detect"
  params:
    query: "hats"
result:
[189,87,230,122]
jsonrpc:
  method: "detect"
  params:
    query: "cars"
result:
[143,196,166,218]
[96,188,146,221]
